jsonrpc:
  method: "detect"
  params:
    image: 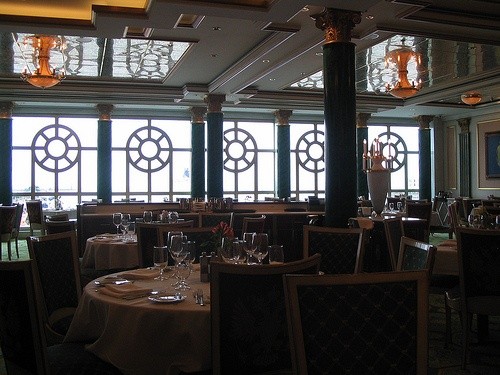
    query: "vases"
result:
[217,246,231,258]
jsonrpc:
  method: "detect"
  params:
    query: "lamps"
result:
[386,38,422,98]
[460,94,482,105]
[363,136,393,221]
[21,33,67,89]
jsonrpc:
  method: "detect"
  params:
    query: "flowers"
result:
[202,220,235,248]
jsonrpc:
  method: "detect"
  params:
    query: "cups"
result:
[143,212,152,223]
[268,245,284,264]
[221,237,247,264]
[168,213,177,223]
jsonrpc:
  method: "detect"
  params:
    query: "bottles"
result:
[199,251,218,282]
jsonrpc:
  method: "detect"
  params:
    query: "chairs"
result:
[0,193,500,375]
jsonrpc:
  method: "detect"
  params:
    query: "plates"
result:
[148,293,187,304]
[94,278,133,286]
[93,233,137,244]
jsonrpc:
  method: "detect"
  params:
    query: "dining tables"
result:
[81,233,138,271]
[134,220,192,264]
[178,209,235,227]
[61,262,283,375]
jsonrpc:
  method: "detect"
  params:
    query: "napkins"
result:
[119,268,159,280]
[100,281,154,300]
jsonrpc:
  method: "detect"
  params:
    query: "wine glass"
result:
[383,202,402,213]
[251,234,268,264]
[113,213,136,241]
[152,231,196,290]
[243,233,257,264]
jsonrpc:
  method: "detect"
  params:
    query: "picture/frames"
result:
[476,118,500,190]
